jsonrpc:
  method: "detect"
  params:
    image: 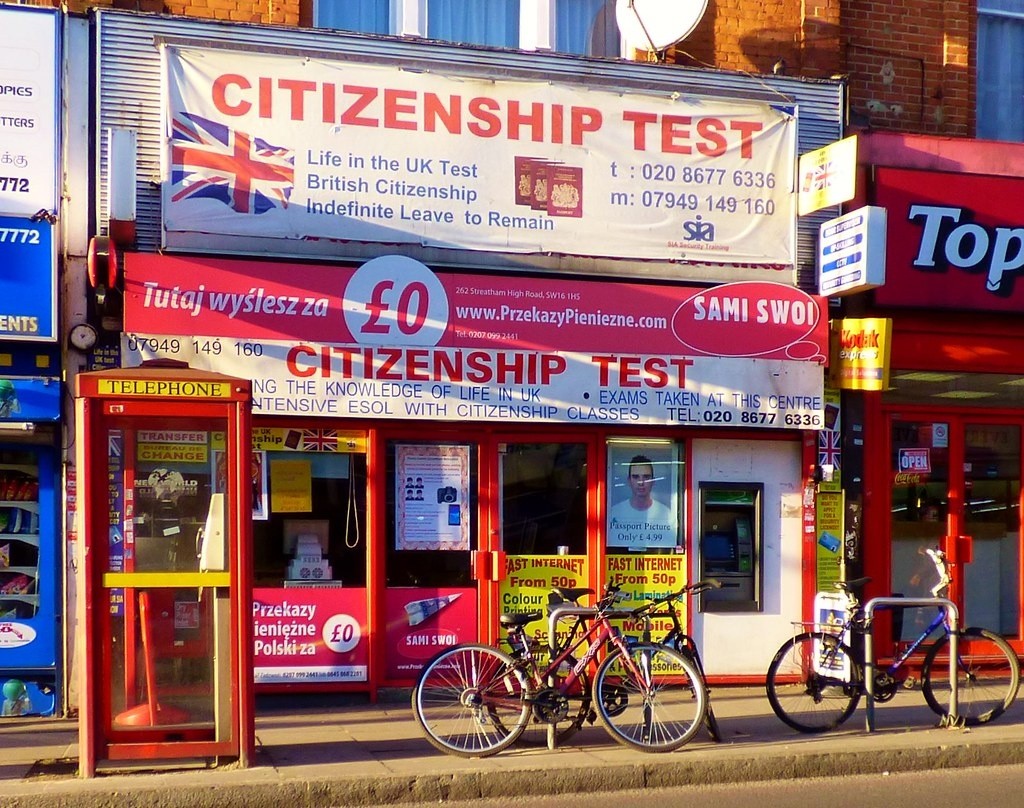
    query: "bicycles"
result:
[412,576,706,758]
[765,545,1020,734]
[485,579,740,743]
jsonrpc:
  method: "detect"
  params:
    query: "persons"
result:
[611,455,672,544]
[415,477,424,489]
[405,477,414,488]
[415,489,424,500]
[406,489,414,500]
